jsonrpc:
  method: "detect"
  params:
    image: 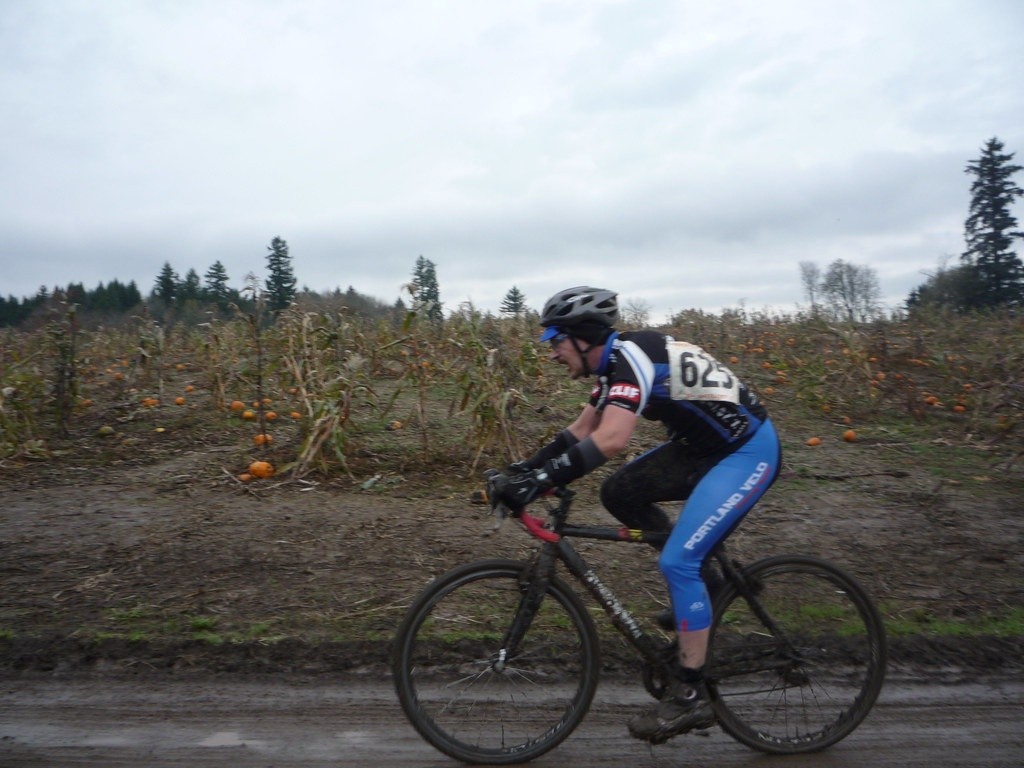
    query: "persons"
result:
[483,285,784,745]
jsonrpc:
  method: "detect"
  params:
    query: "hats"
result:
[540,326,558,343]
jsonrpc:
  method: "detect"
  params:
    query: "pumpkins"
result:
[106,343,973,480]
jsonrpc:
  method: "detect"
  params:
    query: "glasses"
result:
[549,334,569,346]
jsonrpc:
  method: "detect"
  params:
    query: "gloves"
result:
[484,460,552,518]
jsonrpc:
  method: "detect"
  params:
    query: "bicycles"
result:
[383,472,889,767]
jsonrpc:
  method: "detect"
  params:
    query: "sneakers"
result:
[655,610,680,634]
[626,663,721,743]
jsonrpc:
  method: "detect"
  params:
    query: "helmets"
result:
[536,285,622,348]
